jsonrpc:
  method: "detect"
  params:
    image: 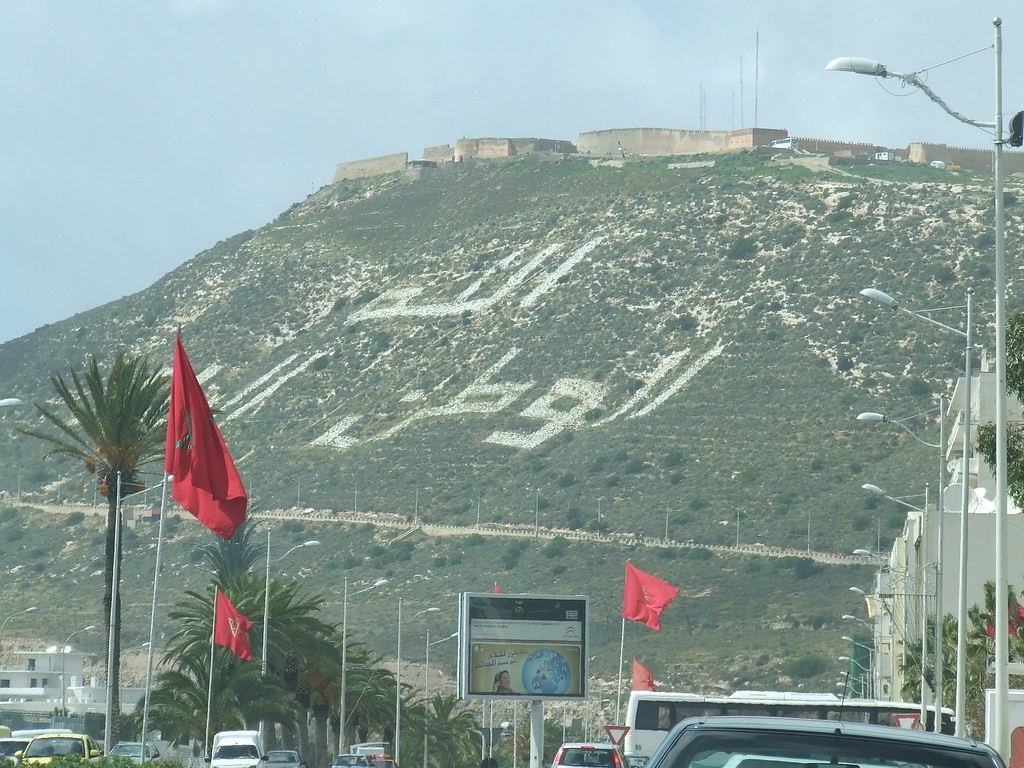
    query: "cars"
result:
[104,740,163,767]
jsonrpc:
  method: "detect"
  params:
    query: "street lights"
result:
[255,527,323,764]
[858,479,930,731]
[420,627,461,768]
[59,622,96,718]
[838,682,865,698]
[840,671,869,698]
[847,584,895,702]
[337,573,390,758]
[852,546,907,702]
[841,634,873,699]
[827,13,1011,768]
[393,595,443,768]
[842,614,883,700]
[857,390,944,735]
[855,268,974,738]
[116,640,151,714]
[838,656,870,699]
[0,605,39,649]
[103,469,176,767]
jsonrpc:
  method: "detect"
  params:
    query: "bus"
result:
[623,686,958,768]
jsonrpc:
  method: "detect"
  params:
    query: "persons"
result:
[493,671,521,695]
[69,742,84,758]
[40,743,56,756]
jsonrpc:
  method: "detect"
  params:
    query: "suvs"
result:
[255,749,308,768]
[328,753,373,768]
[642,714,1006,768]
[370,756,400,768]
[15,732,105,768]
[548,741,631,768]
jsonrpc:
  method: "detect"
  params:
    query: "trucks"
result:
[205,728,270,767]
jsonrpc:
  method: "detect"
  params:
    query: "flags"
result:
[213,591,254,663]
[1005,597,1024,621]
[164,332,249,541]
[986,616,1018,639]
[623,561,680,631]
[629,656,657,693]
